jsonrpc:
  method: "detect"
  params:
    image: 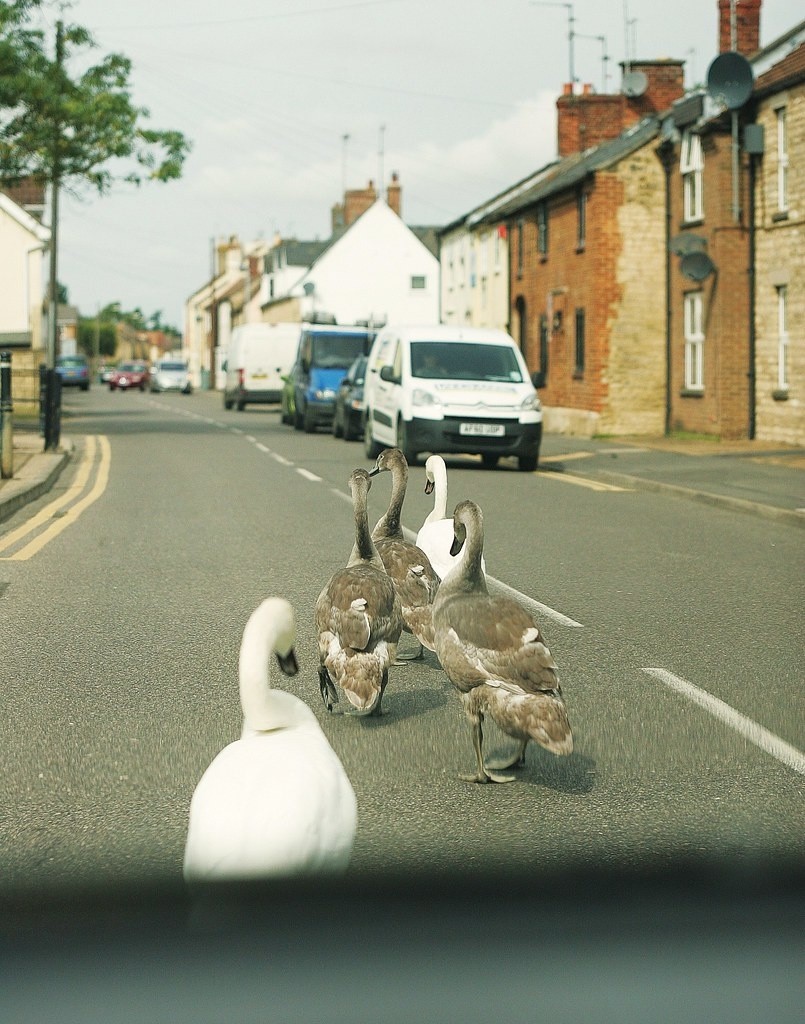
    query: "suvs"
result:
[332,353,369,441]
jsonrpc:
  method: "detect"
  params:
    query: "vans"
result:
[362,322,547,473]
[221,321,304,412]
[294,325,378,434]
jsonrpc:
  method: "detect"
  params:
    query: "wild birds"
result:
[434,498,575,783]
[313,469,402,719]
[183,596,359,878]
[369,448,486,660]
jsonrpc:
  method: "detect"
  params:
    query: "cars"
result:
[149,360,192,395]
[109,359,151,392]
[56,355,90,392]
[100,363,117,383]
[279,363,298,426]
